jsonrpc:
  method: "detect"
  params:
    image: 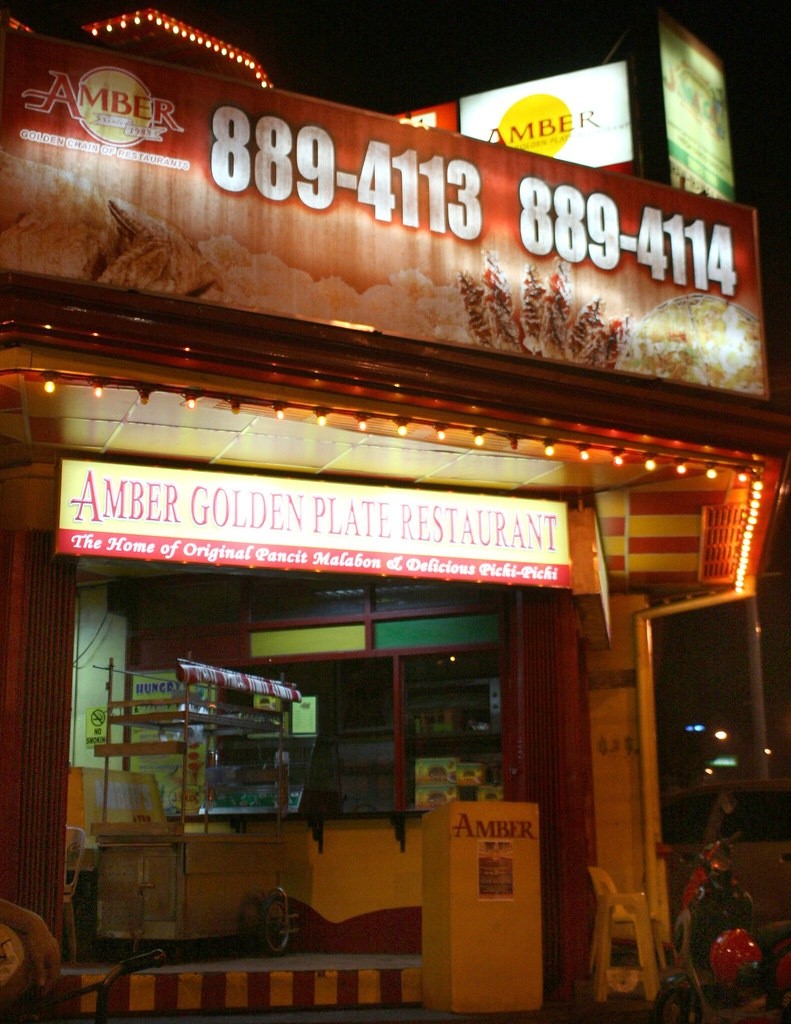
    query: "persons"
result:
[0,892,63,999]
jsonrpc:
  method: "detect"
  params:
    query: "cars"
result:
[660,778,791,929]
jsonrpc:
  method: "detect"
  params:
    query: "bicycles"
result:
[0,949,165,1024]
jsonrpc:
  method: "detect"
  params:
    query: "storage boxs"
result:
[413,756,504,807]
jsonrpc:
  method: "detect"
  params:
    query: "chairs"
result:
[63,827,86,969]
[587,866,663,1001]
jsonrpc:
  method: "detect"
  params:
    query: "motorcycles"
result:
[653,830,791,1024]
